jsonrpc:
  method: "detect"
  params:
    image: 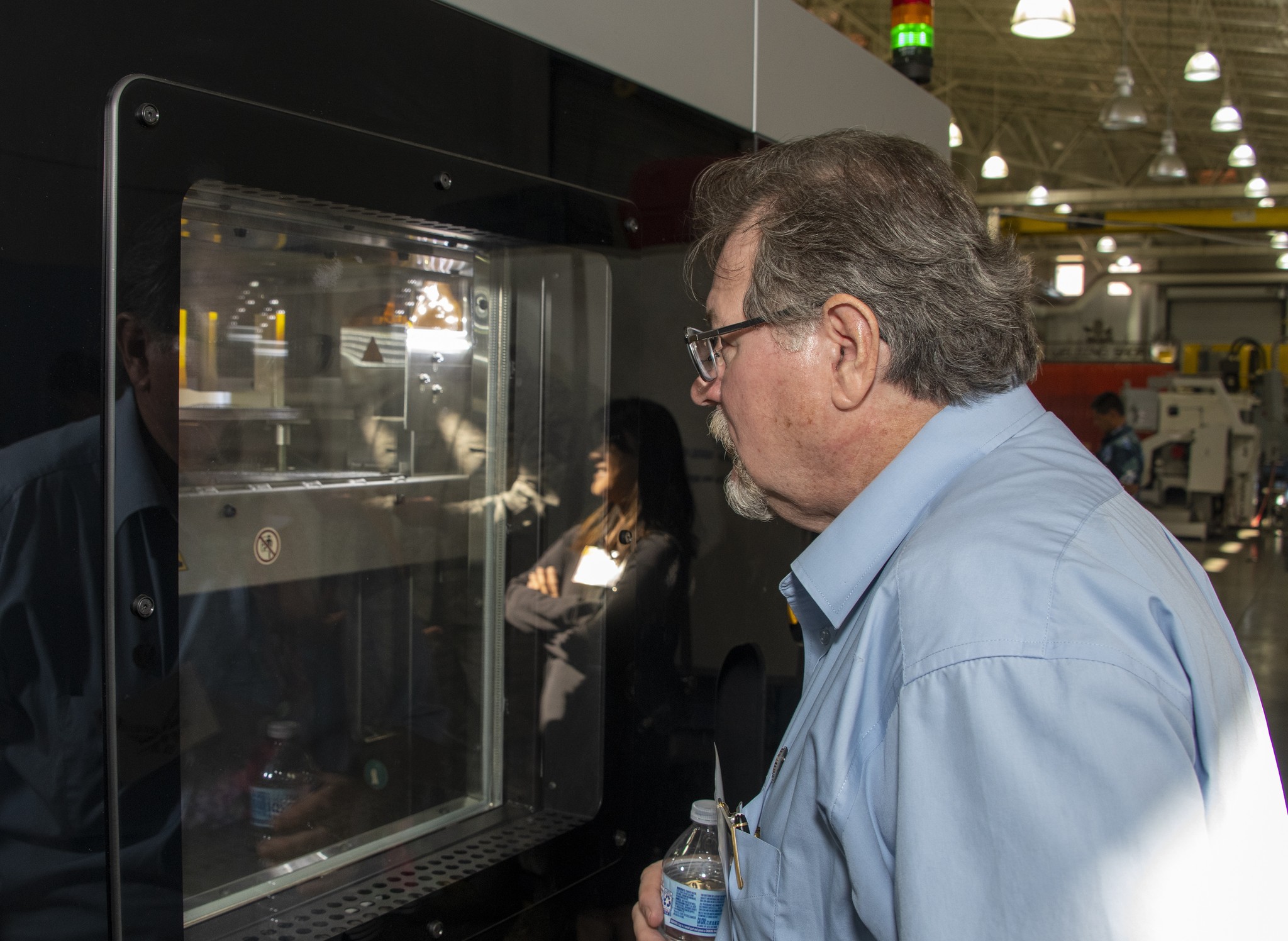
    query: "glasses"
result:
[683,305,798,382]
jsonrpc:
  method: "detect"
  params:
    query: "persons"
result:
[0,231,384,940]
[627,109,1288,941]
[1086,389,1142,500]
[507,395,710,938]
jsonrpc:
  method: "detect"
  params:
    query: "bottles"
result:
[253,720,308,838]
[658,797,726,941]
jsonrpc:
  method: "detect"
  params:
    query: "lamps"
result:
[950,0,1288,303]
